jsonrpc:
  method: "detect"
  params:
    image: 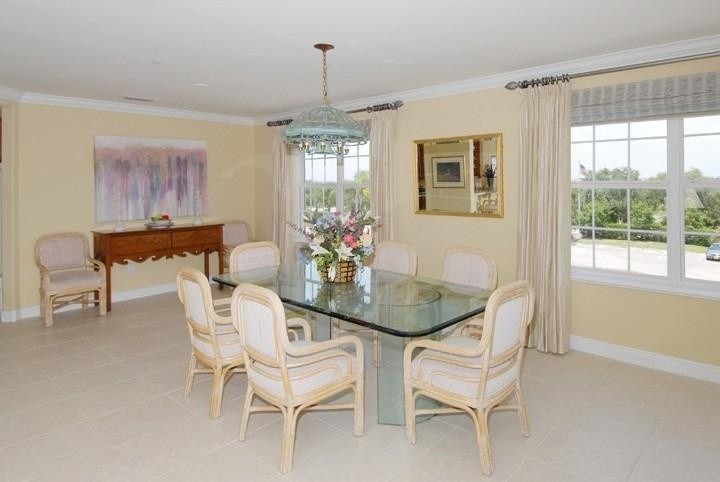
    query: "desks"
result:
[91,224,223,311]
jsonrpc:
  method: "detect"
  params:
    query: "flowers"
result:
[284,200,385,282]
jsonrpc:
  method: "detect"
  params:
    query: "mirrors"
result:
[413,133,504,218]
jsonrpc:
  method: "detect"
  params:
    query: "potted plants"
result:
[482,163,497,191]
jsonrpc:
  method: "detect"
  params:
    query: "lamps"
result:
[282,44,368,157]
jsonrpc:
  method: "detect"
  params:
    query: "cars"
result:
[705,242,720,262]
[572,224,583,243]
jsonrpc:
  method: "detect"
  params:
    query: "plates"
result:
[144,221,174,229]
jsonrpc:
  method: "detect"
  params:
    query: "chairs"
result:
[34,233,107,327]
[222,222,255,273]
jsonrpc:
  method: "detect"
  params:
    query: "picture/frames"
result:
[431,156,465,188]
